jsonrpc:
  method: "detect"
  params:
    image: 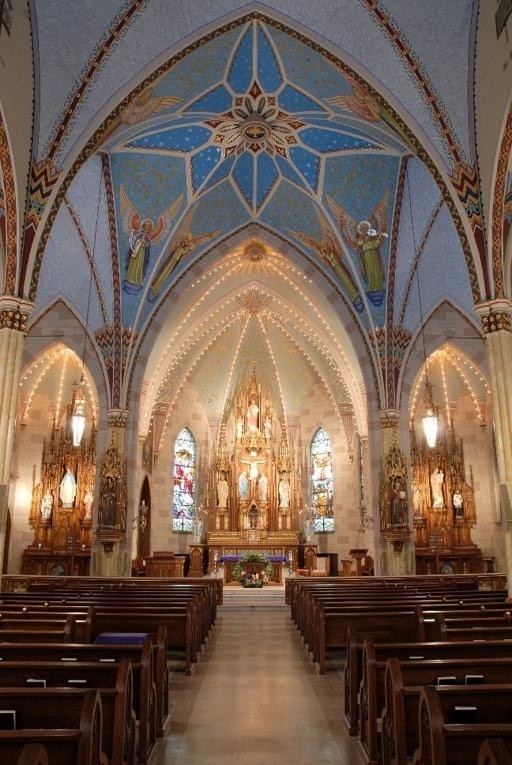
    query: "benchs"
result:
[0,582,216,764]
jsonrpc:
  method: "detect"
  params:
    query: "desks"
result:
[220,555,287,587]
[142,556,186,578]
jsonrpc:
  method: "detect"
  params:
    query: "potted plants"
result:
[232,554,273,588]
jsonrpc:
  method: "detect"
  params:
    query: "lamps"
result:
[403,162,438,448]
[71,154,104,447]
